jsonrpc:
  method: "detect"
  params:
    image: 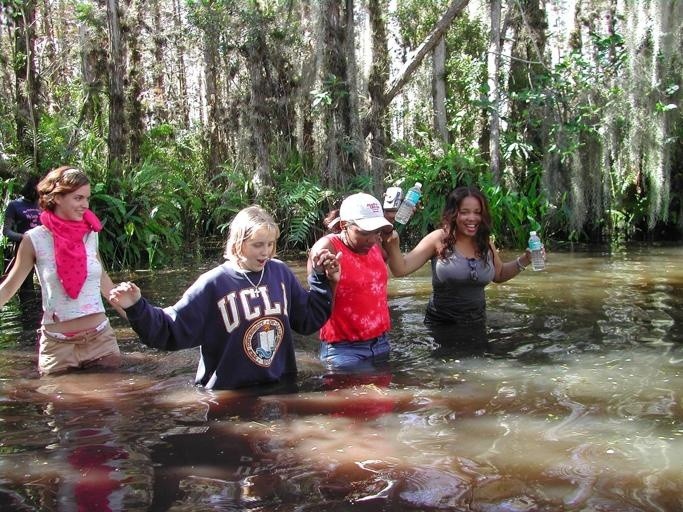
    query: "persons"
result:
[112,201,336,390]
[306,191,423,381]
[1,173,44,296]
[381,185,547,334]
[0,165,129,374]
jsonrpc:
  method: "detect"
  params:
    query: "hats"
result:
[340,192,394,234]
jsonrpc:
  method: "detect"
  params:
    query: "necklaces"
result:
[235,259,265,300]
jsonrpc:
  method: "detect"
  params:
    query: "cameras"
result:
[383,186,403,212]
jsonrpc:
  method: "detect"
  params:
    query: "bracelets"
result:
[517,254,529,272]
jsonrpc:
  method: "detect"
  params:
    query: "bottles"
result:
[394,180,423,226]
[527,229,545,274]
